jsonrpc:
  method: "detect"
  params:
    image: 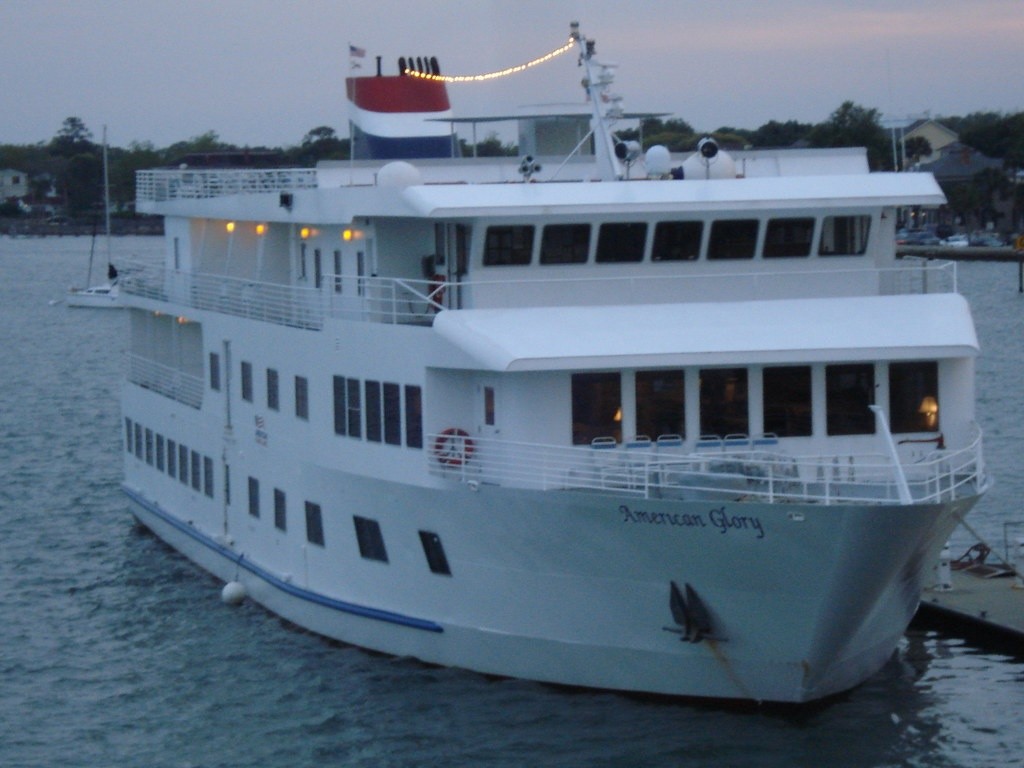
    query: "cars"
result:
[939,235,968,247]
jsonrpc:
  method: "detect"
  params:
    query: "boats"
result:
[113,18,996,705]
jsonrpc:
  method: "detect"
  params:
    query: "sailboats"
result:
[67,122,123,309]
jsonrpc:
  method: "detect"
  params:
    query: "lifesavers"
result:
[433,429,476,466]
